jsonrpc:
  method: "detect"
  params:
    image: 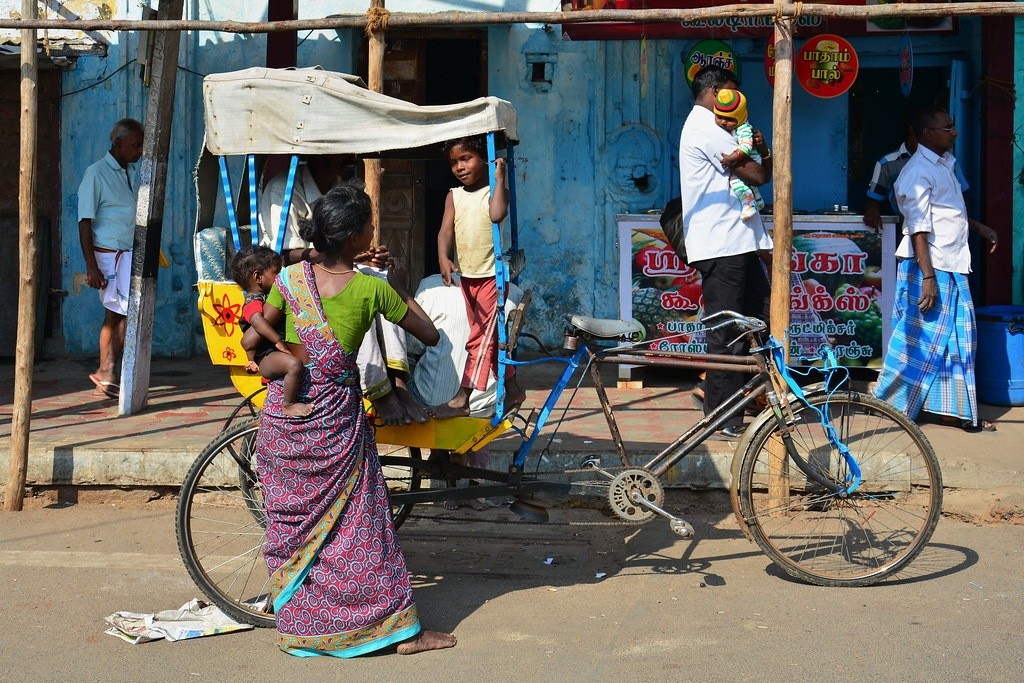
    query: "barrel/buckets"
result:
[972,305,1024,406]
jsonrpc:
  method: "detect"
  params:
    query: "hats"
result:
[713,89,748,128]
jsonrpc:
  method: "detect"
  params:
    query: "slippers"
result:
[706,424,746,442]
[940,416,996,433]
[89,373,120,396]
[863,408,890,420]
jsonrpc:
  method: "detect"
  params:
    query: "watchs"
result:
[763,148,772,160]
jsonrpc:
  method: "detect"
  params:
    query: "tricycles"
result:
[171,305,945,632]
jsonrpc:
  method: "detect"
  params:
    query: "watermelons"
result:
[789,231,866,294]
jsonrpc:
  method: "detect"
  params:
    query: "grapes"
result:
[835,284,882,348]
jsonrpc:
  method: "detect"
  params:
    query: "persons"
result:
[261,153,431,427]
[715,89,765,221]
[863,95,999,435]
[77,120,148,401]
[241,189,457,657]
[678,66,774,437]
[231,244,317,417]
[423,136,526,420]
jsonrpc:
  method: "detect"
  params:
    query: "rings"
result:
[756,138,759,143]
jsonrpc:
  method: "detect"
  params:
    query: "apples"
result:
[802,266,883,302]
[637,243,704,349]
[810,355,882,367]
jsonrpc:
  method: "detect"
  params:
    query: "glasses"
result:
[927,126,958,134]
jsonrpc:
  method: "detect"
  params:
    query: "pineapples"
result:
[631,287,687,334]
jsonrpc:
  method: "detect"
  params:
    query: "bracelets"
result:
[923,275,936,280]
[734,150,742,160]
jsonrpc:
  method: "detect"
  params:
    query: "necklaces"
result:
[315,264,357,276]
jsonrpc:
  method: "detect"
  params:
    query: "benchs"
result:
[200,227,270,400]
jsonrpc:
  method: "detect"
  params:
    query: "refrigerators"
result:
[615,210,900,380]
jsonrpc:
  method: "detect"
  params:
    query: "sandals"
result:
[691,379,706,411]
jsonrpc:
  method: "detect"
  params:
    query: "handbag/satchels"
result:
[659,195,690,266]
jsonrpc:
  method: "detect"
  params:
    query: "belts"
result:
[94,246,130,269]
